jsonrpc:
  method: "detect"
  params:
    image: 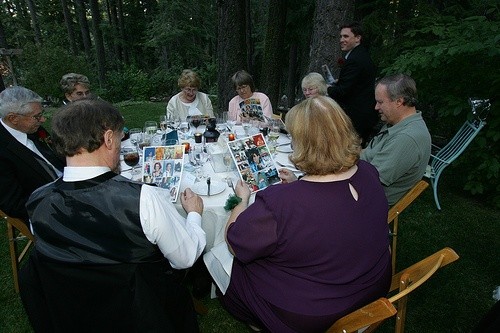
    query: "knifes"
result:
[206,176,211,196]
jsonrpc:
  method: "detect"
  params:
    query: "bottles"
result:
[190,132,204,168]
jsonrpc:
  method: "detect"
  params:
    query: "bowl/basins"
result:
[187,115,209,135]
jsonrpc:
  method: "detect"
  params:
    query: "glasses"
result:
[181,89,199,93]
[302,86,319,93]
[12,109,46,120]
[235,84,249,92]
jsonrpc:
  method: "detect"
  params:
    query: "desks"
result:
[118,124,305,301]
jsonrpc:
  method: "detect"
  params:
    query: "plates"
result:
[275,153,295,168]
[265,133,292,146]
[191,181,227,196]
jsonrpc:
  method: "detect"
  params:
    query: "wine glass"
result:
[123,113,190,181]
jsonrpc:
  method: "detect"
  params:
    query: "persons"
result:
[144,146,184,201]
[326,23,379,146]
[215,94,392,333]
[0,85,66,230]
[228,134,281,192]
[228,70,273,125]
[166,69,215,123]
[359,73,432,209]
[239,97,265,123]
[25,98,207,333]
[59,73,92,102]
[301,73,327,99]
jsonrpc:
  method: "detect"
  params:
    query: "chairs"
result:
[0,209,34,294]
[327,112,486,333]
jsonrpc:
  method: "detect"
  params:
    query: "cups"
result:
[212,110,262,137]
[267,119,280,142]
